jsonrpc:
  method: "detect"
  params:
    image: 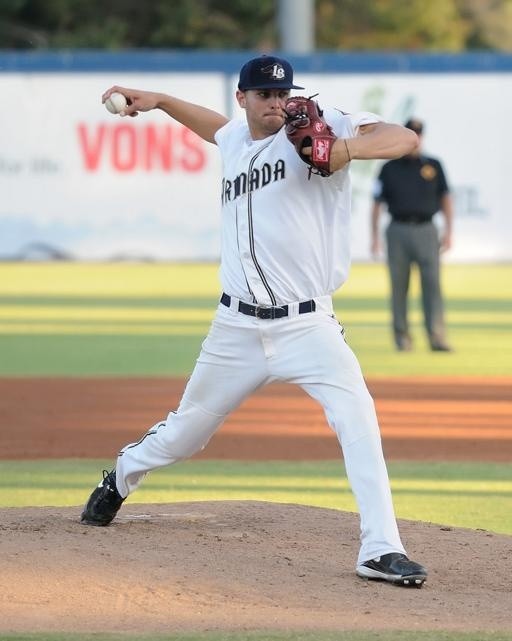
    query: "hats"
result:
[238,53,305,90]
[405,120,423,133]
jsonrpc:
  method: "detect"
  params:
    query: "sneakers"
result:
[80,464,125,526]
[432,342,452,351]
[357,552,427,585]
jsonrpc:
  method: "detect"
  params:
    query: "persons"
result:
[76,53,430,590]
[368,117,455,351]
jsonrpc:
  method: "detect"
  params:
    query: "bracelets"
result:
[343,136,352,163]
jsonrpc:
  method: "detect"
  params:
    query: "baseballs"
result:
[105,92,126,114]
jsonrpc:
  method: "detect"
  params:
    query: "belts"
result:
[391,217,432,225]
[221,293,316,320]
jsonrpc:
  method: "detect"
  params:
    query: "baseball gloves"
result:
[281,93,338,181]
